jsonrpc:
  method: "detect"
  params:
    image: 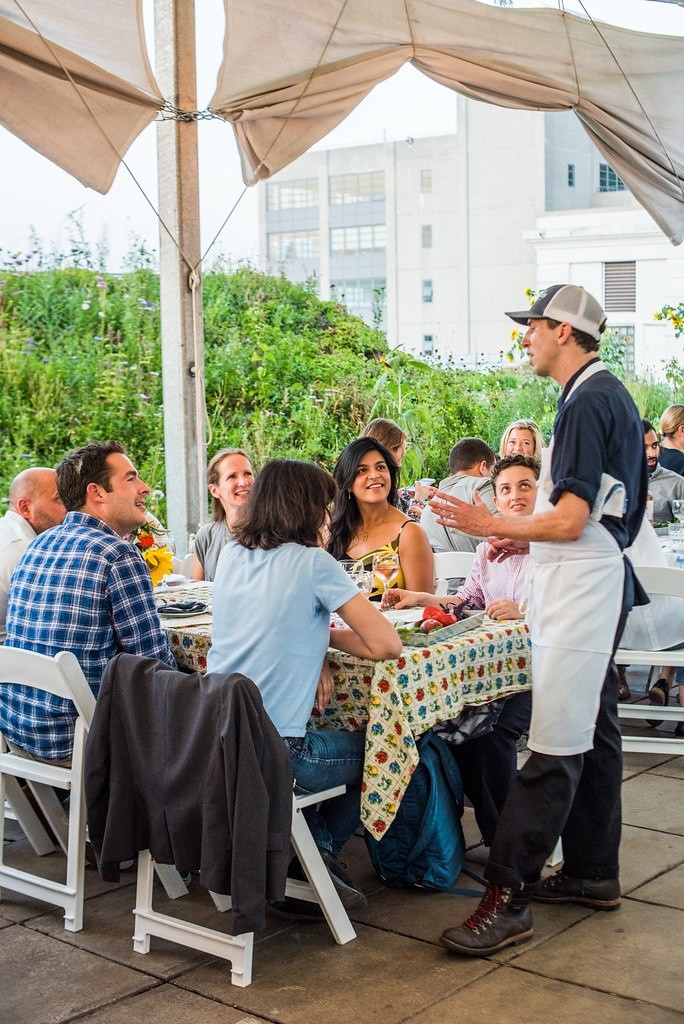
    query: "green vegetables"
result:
[393,620,442,634]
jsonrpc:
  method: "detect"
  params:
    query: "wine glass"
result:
[672,500,684,525]
[373,554,400,612]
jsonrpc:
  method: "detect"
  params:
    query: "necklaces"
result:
[358,501,391,541]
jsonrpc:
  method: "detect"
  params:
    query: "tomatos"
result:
[423,605,457,626]
[420,619,441,632]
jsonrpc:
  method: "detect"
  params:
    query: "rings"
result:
[450,511,453,519]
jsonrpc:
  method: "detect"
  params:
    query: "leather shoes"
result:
[645,678,670,727]
[674,721,684,737]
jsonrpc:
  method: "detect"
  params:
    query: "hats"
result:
[504,284,608,341]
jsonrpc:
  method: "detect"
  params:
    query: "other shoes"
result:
[618,682,631,700]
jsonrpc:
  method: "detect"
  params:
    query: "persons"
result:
[428,284,650,958]
[1,418,546,912]
[615,405,684,736]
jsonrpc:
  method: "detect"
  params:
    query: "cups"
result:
[668,523,683,538]
[347,570,370,599]
[338,559,364,584]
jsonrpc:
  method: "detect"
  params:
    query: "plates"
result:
[155,602,210,618]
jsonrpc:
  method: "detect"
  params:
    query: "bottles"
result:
[644,490,653,524]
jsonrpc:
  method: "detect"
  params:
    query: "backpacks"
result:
[363,726,465,893]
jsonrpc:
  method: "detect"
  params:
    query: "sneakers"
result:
[283,844,370,912]
[440,884,534,956]
[533,870,622,912]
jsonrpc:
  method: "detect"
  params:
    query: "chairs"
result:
[614,566,684,755]
[108,652,357,987]
[0,644,98,932]
[432,551,476,596]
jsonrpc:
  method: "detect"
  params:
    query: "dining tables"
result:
[645,534,684,692]
[149,576,532,886]
